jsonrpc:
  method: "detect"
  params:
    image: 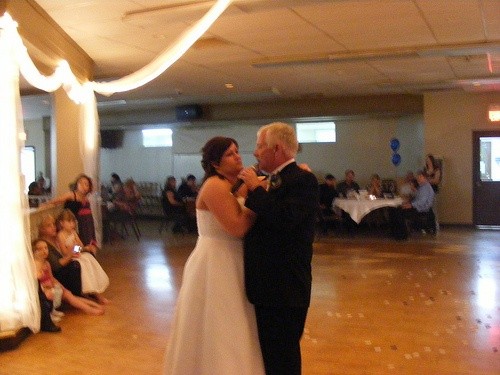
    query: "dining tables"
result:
[333,197,406,238]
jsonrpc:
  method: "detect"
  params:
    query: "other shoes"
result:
[51,308,65,322]
[41,321,61,332]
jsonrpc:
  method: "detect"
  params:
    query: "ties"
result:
[266,172,274,192]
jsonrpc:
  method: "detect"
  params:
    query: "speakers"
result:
[175,105,203,120]
[99,130,117,148]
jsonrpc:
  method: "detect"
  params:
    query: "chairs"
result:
[311,187,439,239]
[160,192,189,233]
[103,199,141,244]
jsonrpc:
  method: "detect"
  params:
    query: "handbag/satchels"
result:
[64,190,83,214]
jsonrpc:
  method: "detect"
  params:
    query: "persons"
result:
[101,170,141,233]
[158,174,198,233]
[238,121,320,375]
[28,174,112,333]
[321,153,442,239]
[164,136,312,375]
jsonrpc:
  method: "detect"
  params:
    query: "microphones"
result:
[231,166,257,192]
